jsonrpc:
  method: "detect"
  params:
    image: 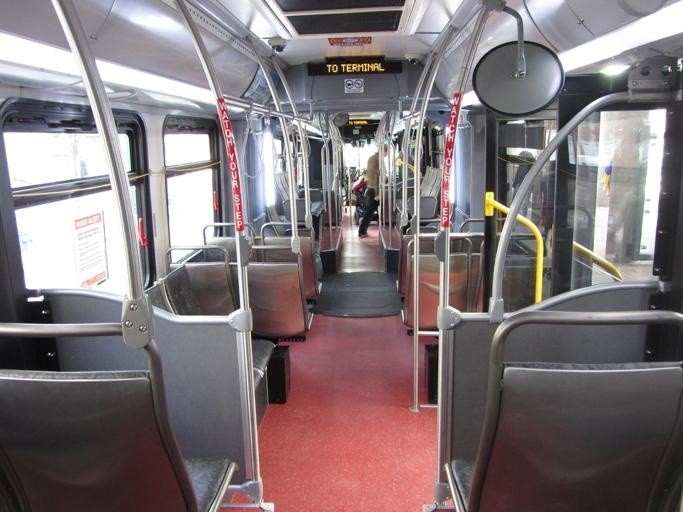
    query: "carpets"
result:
[306,269,405,319]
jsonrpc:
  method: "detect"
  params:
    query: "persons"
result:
[351,172,368,225]
[357,144,389,241]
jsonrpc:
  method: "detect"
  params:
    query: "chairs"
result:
[164,216,323,343]
[0,320,240,512]
[272,170,327,243]
[393,162,443,239]
[441,312,681,511]
[401,216,558,339]
